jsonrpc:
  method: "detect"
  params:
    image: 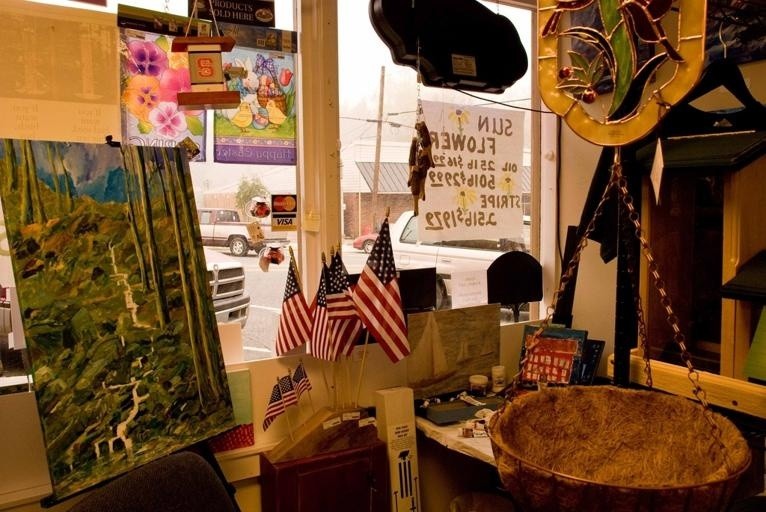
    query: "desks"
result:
[415,414,497,469]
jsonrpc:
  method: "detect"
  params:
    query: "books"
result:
[517,324,606,390]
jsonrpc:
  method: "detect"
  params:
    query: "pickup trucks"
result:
[197,208,291,257]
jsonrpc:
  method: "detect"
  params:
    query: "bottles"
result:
[468,366,506,398]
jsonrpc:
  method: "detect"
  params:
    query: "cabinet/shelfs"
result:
[260,440,388,512]
[629,130,766,421]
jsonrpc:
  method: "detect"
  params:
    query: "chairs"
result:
[66,451,236,512]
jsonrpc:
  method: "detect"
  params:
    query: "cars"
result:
[352,210,531,312]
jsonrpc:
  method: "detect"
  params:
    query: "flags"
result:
[279,375,298,410]
[291,365,312,398]
[262,385,285,432]
[275,218,412,364]
